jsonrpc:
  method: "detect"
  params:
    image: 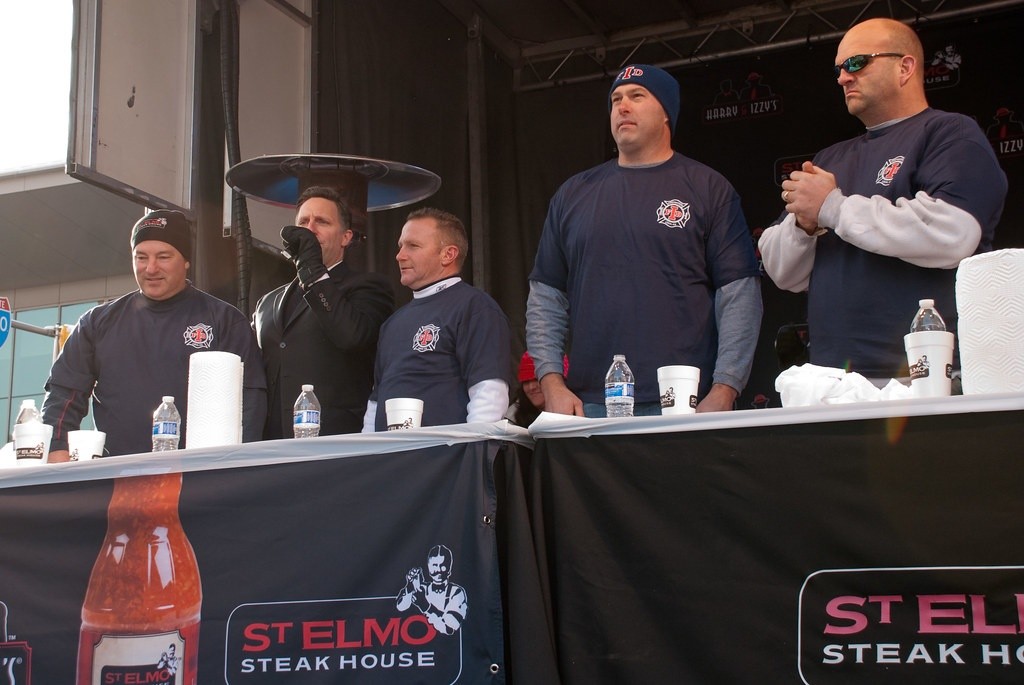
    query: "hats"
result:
[131,210,192,262]
[608,64,680,138]
[519,348,569,380]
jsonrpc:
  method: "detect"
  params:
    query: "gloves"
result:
[280,226,322,271]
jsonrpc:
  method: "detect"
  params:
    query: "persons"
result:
[361,208,510,435]
[759,18,1006,384]
[251,187,393,442]
[42,210,266,463]
[503,350,568,428]
[526,64,762,418]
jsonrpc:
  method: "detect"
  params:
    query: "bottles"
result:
[605,354,634,418]
[293,385,321,438]
[152,396,181,452]
[77,472,202,685]
[13,399,42,455]
[910,299,947,333]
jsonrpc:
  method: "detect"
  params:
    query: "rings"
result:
[783,191,792,203]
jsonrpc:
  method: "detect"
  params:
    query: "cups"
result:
[657,365,700,415]
[68,430,107,461]
[385,398,424,430]
[14,425,53,465]
[904,331,955,399]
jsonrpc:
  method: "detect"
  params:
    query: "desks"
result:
[0,392,1024,685]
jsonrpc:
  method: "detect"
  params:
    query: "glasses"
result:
[830,52,905,80]
